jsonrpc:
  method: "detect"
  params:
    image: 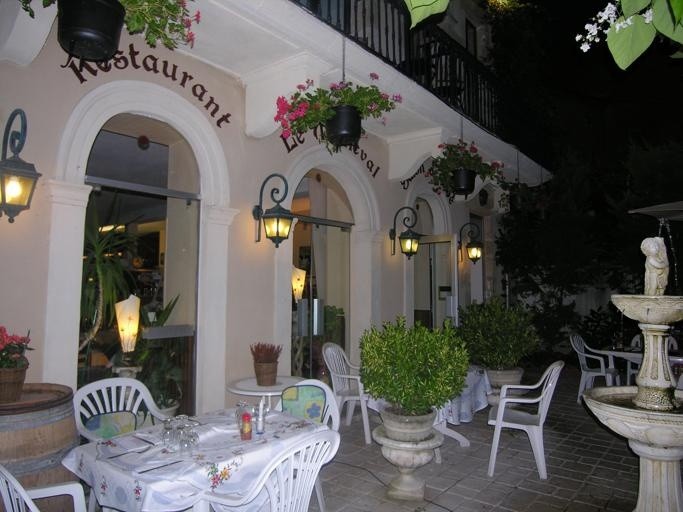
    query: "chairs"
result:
[72,378,171,442]
[322,342,373,444]
[569,333,621,406]
[0,463,87,511]
[486,360,567,479]
[626,333,678,385]
[194,430,340,511]
[273,378,345,511]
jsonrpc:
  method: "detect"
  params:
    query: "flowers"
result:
[272,68,402,138]
[424,139,504,203]
[249,341,283,362]
[497,181,551,216]
[18,1,203,53]
[0,326,35,366]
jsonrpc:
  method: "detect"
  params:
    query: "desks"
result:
[365,366,493,465]
[610,347,683,367]
[226,375,313,411]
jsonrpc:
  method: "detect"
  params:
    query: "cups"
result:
[234,397,266,435]
[162,413,201,458]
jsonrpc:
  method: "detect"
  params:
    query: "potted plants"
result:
[137,335,190,418]
[358,316,471,502]
[456,301,545,408]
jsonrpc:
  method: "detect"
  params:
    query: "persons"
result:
[653,236,671,295]
[639,235,665,296]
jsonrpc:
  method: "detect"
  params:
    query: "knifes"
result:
[137,460,185,478]
[130,433,154,446]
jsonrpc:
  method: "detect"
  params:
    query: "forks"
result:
[102,446,152,460]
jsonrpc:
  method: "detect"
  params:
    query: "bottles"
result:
[239,413,253,440]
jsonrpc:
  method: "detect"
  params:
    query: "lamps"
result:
[291,266,307,377]
[457,222,485,266]
[388,206,421,261]
[0,109,42,224]
[111,294,141,410]
[250,173,295,248]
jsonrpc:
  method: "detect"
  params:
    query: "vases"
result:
[253,361,279,386]
[509,193,527,213]
[56,1,126,63]
[0,356,31,403]
[453,169,476,195]
[326,105,361,147]
